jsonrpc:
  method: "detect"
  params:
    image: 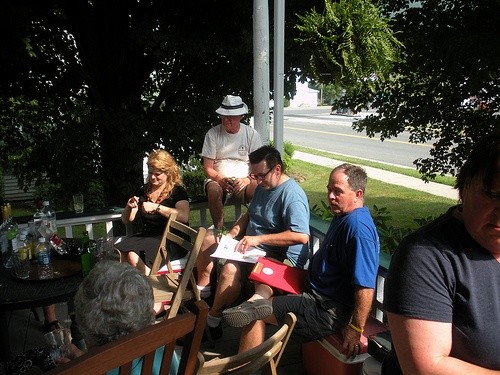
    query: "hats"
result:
[215,95,248,116]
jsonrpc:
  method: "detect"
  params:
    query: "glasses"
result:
[249,165,275,180]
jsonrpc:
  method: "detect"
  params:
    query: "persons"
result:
[41,258,181,375]
[199,95,263,229]
[381,131,500,375]
[113,149,190,276]
[222,163,380,367]
[176,144,311,346]
[42,300,64,333]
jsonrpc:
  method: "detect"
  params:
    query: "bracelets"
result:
[349,322,365,333]
[156,204,162,212]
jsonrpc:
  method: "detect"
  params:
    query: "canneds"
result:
[229,177,237,193]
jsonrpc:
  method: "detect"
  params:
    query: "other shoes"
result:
[223,299,273,328]
[183,292,213,312]
[71,339,87,352]
[176,320,223,346]
[42,320,66,334]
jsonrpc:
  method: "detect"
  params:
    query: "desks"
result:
[0,237,120,356]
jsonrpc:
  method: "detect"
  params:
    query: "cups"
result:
[73,193,84,214]
[96,237,114,262]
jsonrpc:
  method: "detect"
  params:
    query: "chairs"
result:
[194,310,298,375]
[42,296,210,375]
[151,210,212,321]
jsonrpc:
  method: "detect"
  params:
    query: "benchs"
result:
[300,311,387,375]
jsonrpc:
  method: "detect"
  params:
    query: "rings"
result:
[242,242,245,245]
[355,345,359,346]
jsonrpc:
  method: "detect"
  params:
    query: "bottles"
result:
[41,200,59,237]
[79,231,94,277]
[35,237,53,280]
[11,225,32,280]
[0,201,18,268]
[33,208,48,229]
